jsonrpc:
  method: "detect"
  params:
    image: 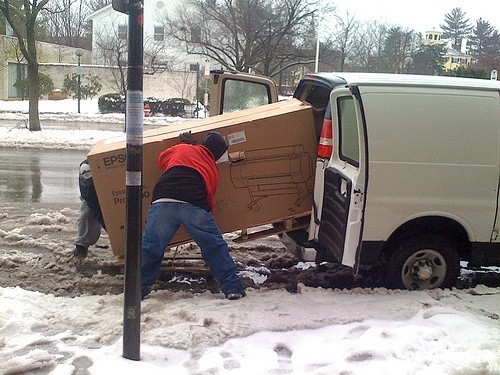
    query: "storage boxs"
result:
[86,97,319,258]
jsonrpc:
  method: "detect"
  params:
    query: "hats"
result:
[80,164,93,186]
[203,131,228,161]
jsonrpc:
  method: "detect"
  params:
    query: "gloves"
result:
[179,130,195,146]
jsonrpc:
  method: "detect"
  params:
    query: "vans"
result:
[208,70,500,290]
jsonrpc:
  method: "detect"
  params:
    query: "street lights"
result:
[75,48,84,114]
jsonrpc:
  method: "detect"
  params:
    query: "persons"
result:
[68,158,108,261]
[139,132,247,302]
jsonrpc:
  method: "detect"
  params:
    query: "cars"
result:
[146,97,165,114]
[99,93,154,116]
[160,98,192,116]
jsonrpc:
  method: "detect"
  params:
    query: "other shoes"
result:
[74,245,87,258]
[228,293,242,300]
